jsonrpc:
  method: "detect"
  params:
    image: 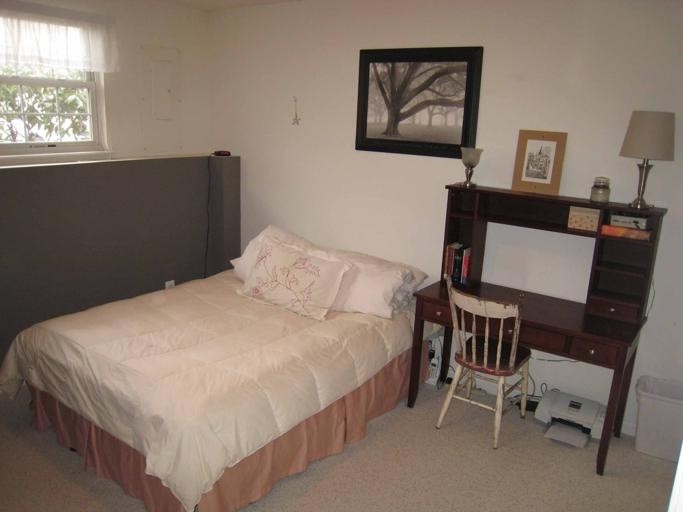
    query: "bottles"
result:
[589,177,610,203]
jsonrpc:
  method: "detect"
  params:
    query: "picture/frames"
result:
[354,46,483,159]
[509,129,568,196]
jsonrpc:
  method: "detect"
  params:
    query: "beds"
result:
[29,271,442,510]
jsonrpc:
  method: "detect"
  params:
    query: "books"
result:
[608,214,649,231]
[599,224,652,242]
[442,241,471,289]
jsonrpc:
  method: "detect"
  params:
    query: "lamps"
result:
[618,110,675,210]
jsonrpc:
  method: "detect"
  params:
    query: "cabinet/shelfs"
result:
[440,181,669,327]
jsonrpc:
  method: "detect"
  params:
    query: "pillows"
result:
[234,231,354,321]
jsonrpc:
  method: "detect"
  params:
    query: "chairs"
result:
[434,276,532,450]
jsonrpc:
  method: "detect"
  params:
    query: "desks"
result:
[406,281,648,477]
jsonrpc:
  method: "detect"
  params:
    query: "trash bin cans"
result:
[634,375,683,463]
